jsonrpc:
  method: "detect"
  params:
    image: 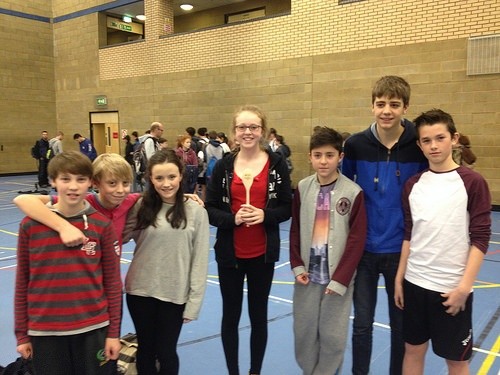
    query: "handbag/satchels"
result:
[45,147,53,159]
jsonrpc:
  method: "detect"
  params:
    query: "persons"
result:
[339,74,429,375]
[123,121,292,205]
[33,130,49,187]
[206,105,292,375]
[453,135,478,172]
[47,132,64,160]
[122,150,209,375]
[14,153,206,250]
[289,125,367,375]
[394,108,492,375]
[13,150,123,374]
[73,133,97,163]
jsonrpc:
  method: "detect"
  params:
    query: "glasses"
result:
[235,125,262,132]
[157,128,163,132]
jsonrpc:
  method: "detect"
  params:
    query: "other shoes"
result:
[40,184,51,188]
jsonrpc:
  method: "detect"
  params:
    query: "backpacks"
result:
[32,140,43,159]
[132,137,156,175]
[201,138,209,152]
